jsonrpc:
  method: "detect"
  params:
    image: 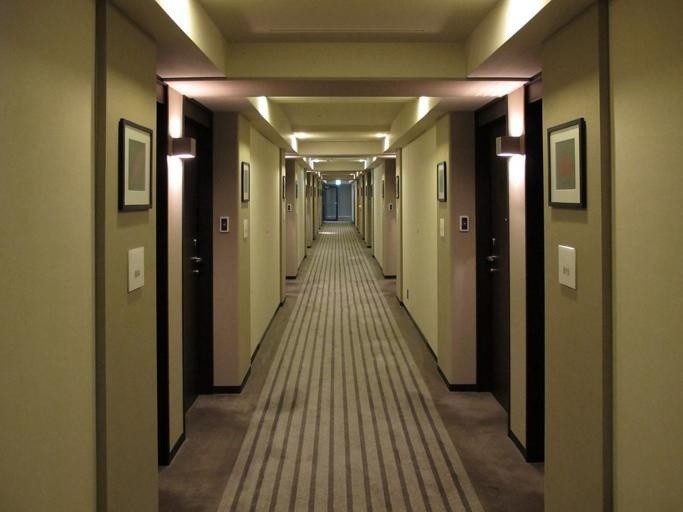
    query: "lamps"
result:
[496,136,521,158]
[172,137,198,159]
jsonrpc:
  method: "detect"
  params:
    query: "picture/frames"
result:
[547,117,587,210]
[118,117,152,211]
[242,161,253,202]
[279,176,324,198]
[352,178,400,200]
[437,161,447,202]
